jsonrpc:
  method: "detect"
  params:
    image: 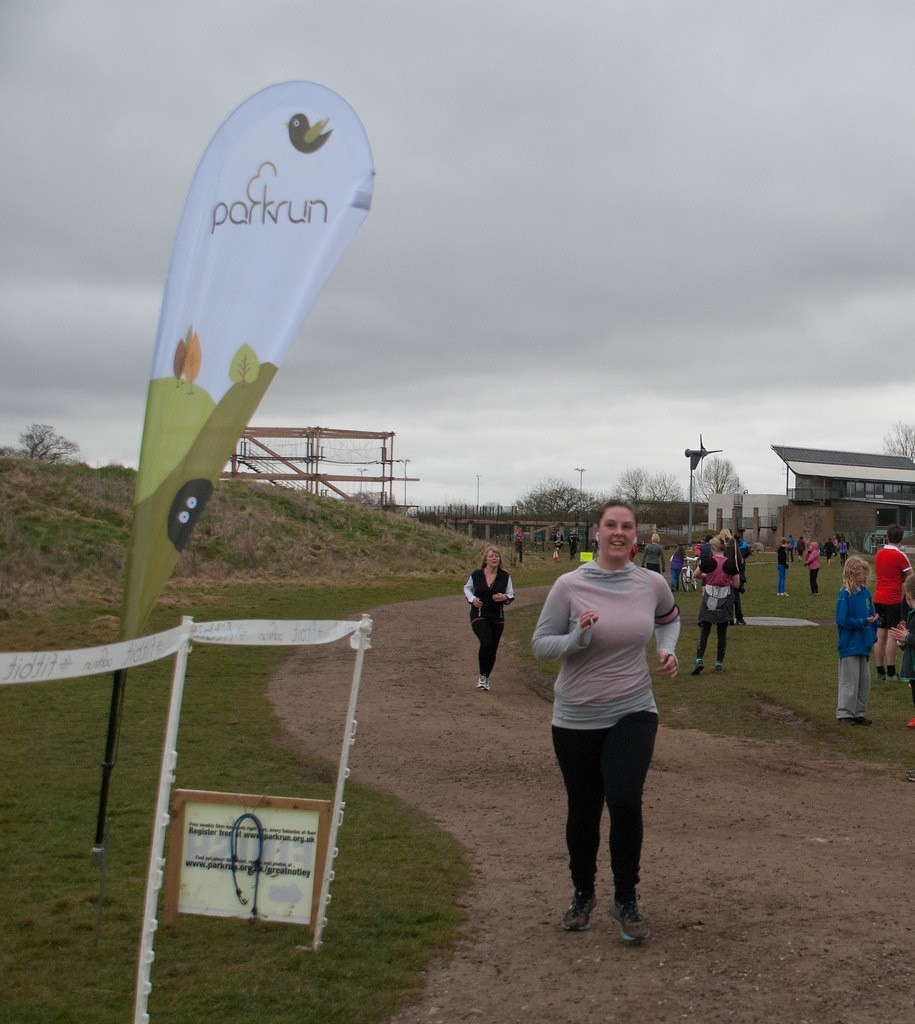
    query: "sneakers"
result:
[476,675,487,688]
[610,894,649,940]
[562,892,598,931]
[481,680,491,690]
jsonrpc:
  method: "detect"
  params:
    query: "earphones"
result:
[633,537,637,545]
[596,531,599,543]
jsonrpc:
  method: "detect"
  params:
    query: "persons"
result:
[463,547,515,692]
[567,529,579,560]
[835,556,878,727]
[628,536,638,562]
[692,528,753,626]
[873,522,915,782]
[669,547,685,592]
[787,534,847,566]
[591,532,599,560]
[531,499,680,942]
[641,534,666,574]
[804,541,821,595]
[553,530,564,558]
[777,538,790,597]
[513,526,524,563]
[691,536,740,675]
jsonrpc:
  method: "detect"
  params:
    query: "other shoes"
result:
[776,592,790,596]
[853,717,872,726]
[837,717,857,725]
[810,592,821,597]
[729,620,735,625]
[736,618,746,625]
[885,673,910,683]
[877,671,887,680]
[715,665,722,672]
[906,718,915,728]
[691,660,704,675]
[905,771,915,782]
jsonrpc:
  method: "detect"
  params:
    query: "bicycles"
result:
[681,557,701,592]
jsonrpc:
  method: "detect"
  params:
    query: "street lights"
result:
[574,467,586,522]
[476,474,480,505]
[356,468,369,493]
